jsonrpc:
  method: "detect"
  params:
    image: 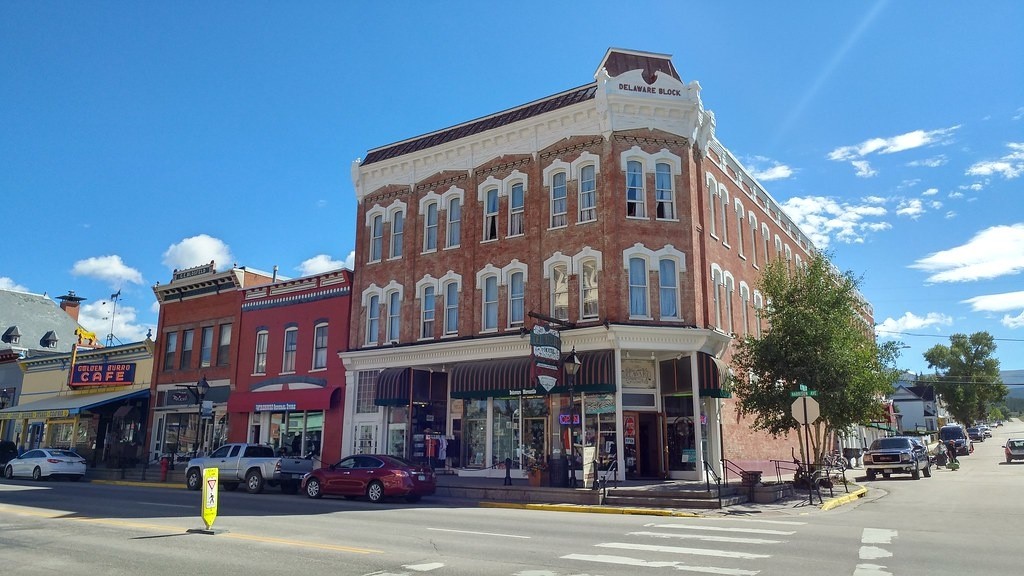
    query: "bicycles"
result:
[815,449,849,472]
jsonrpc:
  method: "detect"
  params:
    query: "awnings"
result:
[696,352,733,398]
[374,367,411,406]
[450,350,616,399]
[0,388,150,420]
[228,386,339,410]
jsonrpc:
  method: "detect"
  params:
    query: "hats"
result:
[424,428,432,433]
[950,440,955,442]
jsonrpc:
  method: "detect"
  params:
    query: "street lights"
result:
[189,374,211,459]
[563,345,581,488]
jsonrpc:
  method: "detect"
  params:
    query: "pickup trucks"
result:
[861,436,933,480]
[185,444,314,494]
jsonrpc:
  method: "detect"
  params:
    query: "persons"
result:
[936,439,956,471]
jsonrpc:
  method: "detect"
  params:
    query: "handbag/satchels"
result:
[946,458,959,469]
[932,447,939,455]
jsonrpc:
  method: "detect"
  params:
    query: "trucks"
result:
[940,425,974,456]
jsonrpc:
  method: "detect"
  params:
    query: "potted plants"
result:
[523,428,550,487]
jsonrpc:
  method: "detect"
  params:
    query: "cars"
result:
[967,428,985,442]
[4,447,87,480]
[996,419,1003,426]
[977,425,989,430]
[981,428,992,438]
[300,453,437,503]
[1001,438,1024,463]
[0,439,19,479]
[989,422,998,428]
